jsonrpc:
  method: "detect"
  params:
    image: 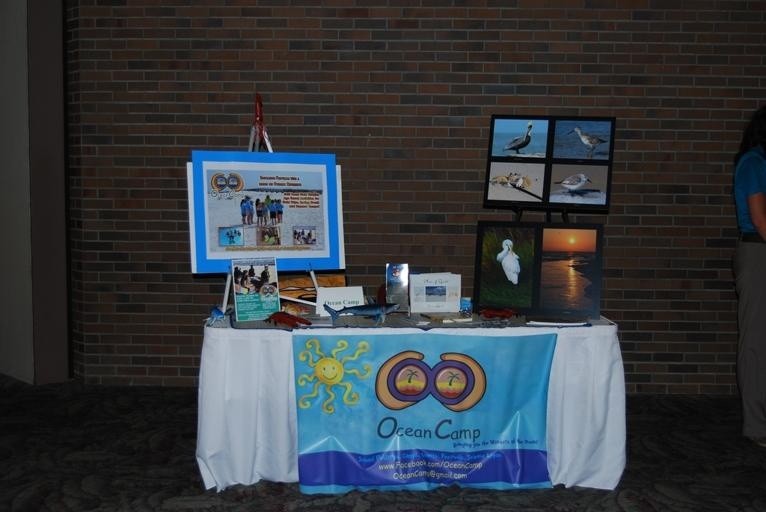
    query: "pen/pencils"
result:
[421,314,431,319]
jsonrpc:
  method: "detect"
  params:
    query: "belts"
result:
[736,231,766,243]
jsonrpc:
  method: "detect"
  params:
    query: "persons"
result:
[734,103,765,448]
[234,264,270,290]
[294,228,316,244]
[240,195,284,228]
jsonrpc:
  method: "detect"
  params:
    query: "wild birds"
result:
[489,172,533,190]
[495,238,521,287]
[566,126,608,157]
[553,172,593,197]
[502,121,534,155]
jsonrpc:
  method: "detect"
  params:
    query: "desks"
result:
[205,301,619,488]
